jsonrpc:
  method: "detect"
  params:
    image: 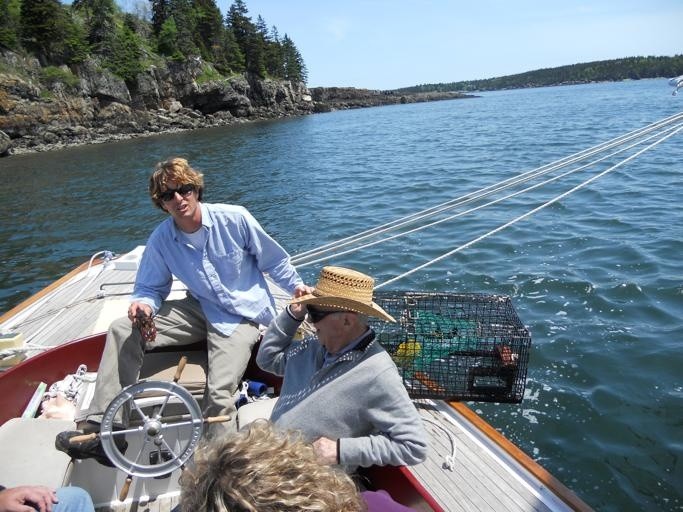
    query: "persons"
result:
[256,266,428,482]
[55,156,316,468]
[170,412,371,512]
[0,480,94,512]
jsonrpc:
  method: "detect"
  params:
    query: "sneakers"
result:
[54,430,130,470]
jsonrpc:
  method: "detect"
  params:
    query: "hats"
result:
[284,264,398,326]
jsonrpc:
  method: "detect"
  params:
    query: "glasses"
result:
[158,183,197,203]
[304,303,346,325]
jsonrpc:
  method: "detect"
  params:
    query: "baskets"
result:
[367,288,533,405]
